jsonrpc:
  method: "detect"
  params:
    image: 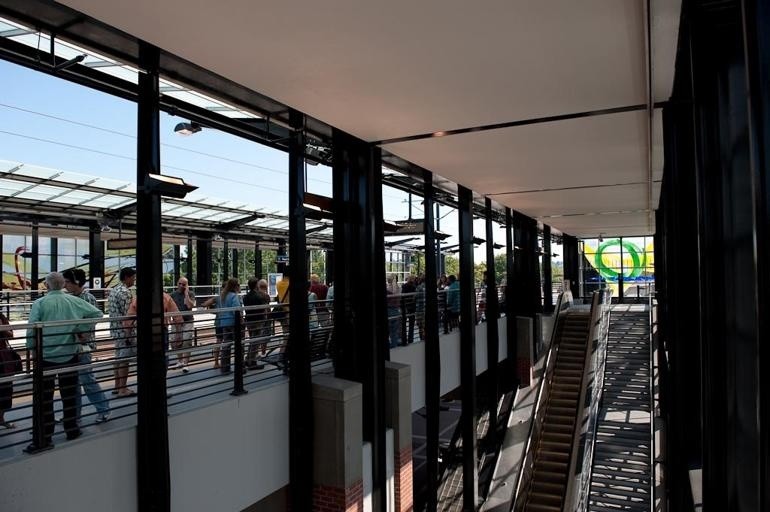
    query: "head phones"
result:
[72,270,80,284]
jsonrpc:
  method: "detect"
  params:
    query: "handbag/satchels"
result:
[270,305,283,320]
[0,350,23,377]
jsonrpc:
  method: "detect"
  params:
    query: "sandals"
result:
[112,388,136,396]
[0,420,15,428]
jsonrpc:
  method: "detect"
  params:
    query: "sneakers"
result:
[247,360,264,370]
[95,410,113,423]
[214,364,221,368]
[176,360,190,373]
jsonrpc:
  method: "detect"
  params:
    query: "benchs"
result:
[260,326,332,375]
